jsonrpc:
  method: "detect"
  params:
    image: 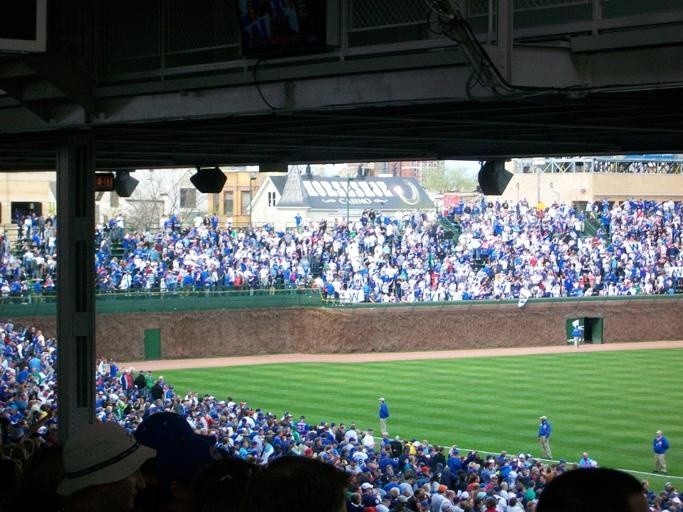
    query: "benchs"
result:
[11,235,141,267]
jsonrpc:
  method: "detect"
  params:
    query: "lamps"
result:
[476,156,513,196]
[110,168,139,198]
[189,166,228,194]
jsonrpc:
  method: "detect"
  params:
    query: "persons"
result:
[652,430,669,473]
[579,452,596,468]
[572,325,581,349]
[377,397,390,438]
[537,415,552,460]
[1,321,682,510]
[0,195,683,303]
